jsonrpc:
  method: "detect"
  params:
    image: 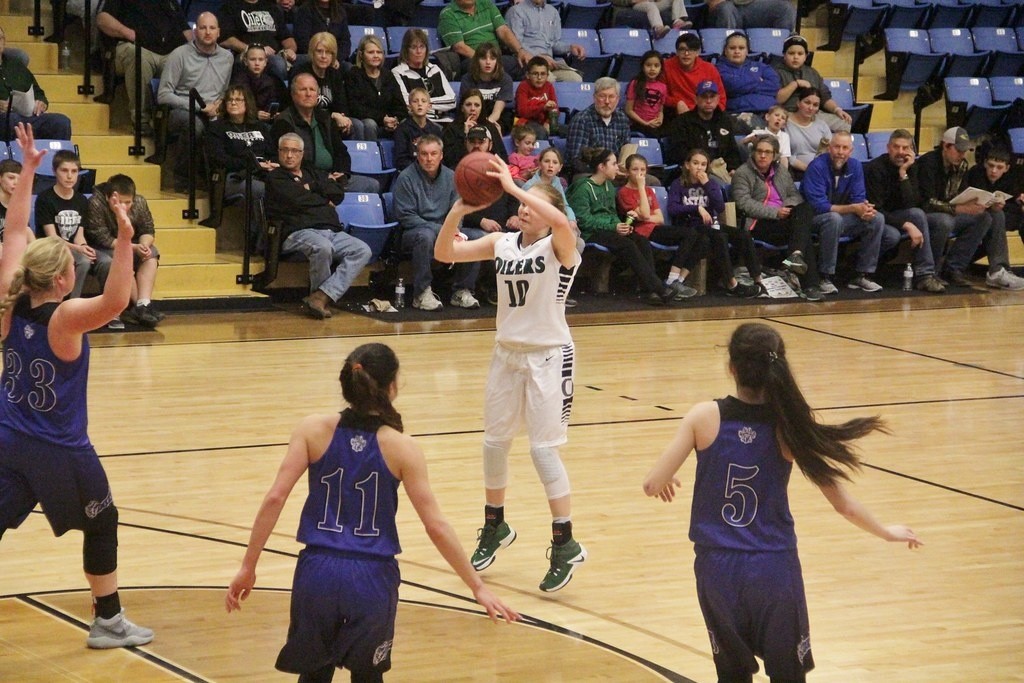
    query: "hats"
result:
[696,80,718,96]
[468,125,493,141]
[943,125,975,152]
[781,32,808,56]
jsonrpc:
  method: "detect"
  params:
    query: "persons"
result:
[0,120,155,649]
[86,173,165,326]
[63,0,1024,319]
[225,342,522,683]
[34,148,125,330]
[643,322,925,683]
[0,159,36,248]
[433,154,588,592]
[0,26,72,142]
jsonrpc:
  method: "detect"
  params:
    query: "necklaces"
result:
[230,119,251,147]
[375,68,378,85]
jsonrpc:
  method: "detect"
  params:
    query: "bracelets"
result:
[900,174,908,180]
[834,107,841,113]
[795,80,799,87]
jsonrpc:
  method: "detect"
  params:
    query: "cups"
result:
[624,216,634,227]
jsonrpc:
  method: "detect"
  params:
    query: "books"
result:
[950,186,1012,208]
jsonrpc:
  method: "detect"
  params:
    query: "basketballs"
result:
[454,151,505,206]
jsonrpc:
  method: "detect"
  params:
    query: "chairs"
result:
[0,0,1024,291]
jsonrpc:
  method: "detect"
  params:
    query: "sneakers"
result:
[109,315,125,329]
[847,272,882,292]
[986,267,1024,291]
[86,606,154,650]
[470,520,517,571]
[412,283,445,311]
[450,288,480,308]
[539,536,588,592]
[817,276,838,294]
[130,303,166,328]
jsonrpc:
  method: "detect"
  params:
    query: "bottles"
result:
[902,263,914,291]
[395,278,405,308]
[549,108,559,136]
[61,40,70,68]
[711,216,720,231]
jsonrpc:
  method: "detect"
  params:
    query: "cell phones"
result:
[269,103,279,118]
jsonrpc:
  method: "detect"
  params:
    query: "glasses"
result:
[278,147,301,155]
[754,149,774,156]
[225,97,244,104]
[528,70,549,79]
[678,47,698,54]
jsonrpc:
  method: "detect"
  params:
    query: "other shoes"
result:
[301,289,332,318]
[673,19,692,30]
[940,268,973,286]
[654,25,670,40]
[482,278,499,305]
[648,269,826,302]
[916,275,945,292]
